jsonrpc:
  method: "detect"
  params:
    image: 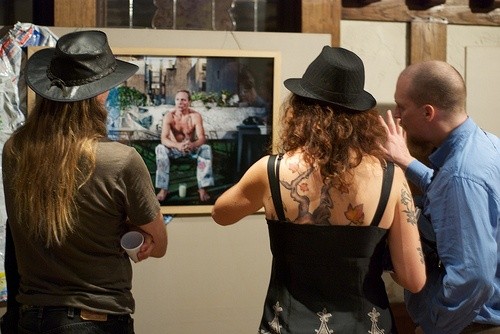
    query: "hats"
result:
[283,45,378,111]
[23,31,139,101]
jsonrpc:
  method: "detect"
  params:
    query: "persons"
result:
[156,90,214,203]
[377,60,500,334]
[210,45,428,334]
[0,31,168,334]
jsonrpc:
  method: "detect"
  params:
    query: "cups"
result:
[121,232,144,262]
